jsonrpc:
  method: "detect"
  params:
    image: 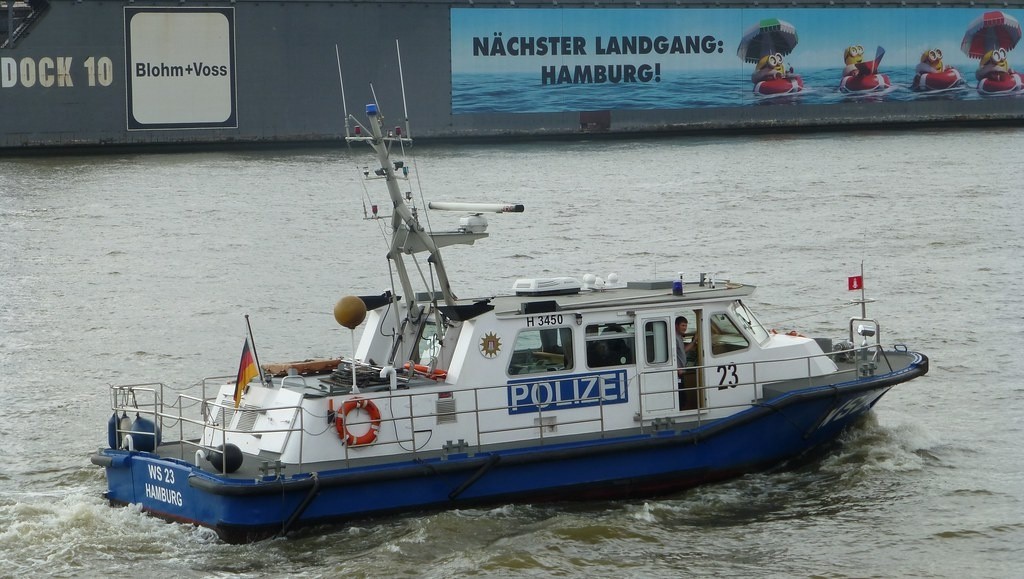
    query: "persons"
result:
[676,316,698,405]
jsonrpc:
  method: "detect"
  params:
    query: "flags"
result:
[234,337,258,408]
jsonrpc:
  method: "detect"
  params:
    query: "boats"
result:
[88,38,930,537]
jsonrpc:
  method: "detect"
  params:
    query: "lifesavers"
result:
[336,395,382,450]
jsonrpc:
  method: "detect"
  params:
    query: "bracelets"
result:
[693,339,696,345]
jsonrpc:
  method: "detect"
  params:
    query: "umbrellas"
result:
[737,18,798,64]
[961,11,1022,59]
